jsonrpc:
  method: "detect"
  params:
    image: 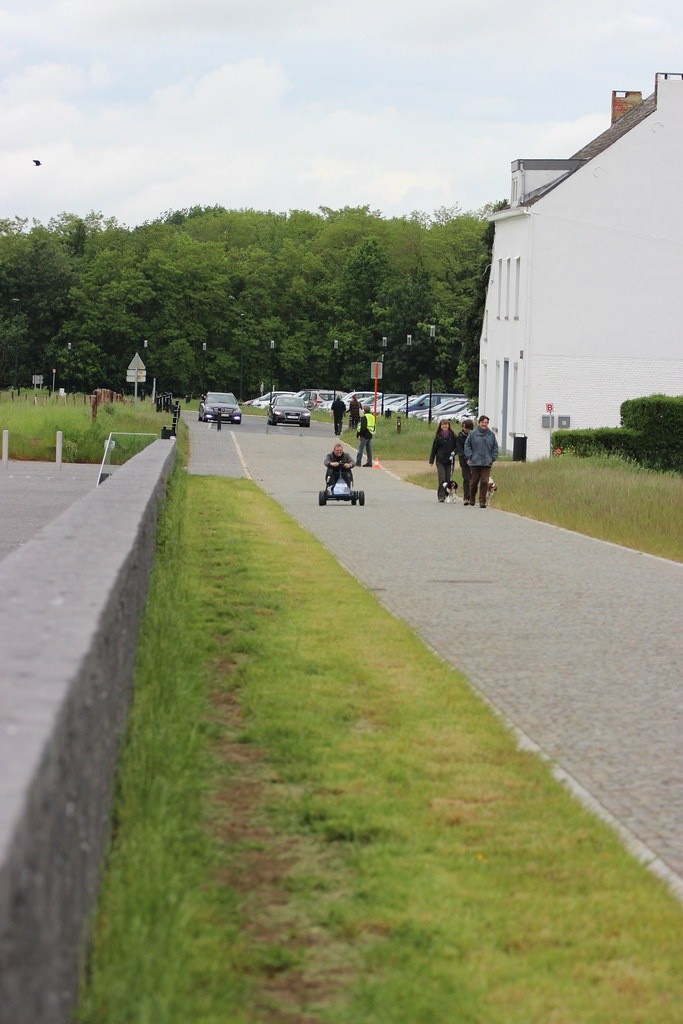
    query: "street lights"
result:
[11,299,24,390]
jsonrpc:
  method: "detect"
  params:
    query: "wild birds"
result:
[32,159,42,166]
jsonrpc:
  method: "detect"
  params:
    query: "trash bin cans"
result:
[385,408,392,419]
[514,433,528,463]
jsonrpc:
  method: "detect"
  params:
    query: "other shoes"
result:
[362,463,372,467]
[438,497,444,502]
[470,499,475,506]
[355,463,360,467]
[463,498,470,505]
[480,502,486,508]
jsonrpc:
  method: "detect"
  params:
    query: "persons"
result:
[323,443,356,495]
[464,415,498,508]
[429,419,458,502]
[355,405,376,467]
[453,420,474,505]
[346,396,361,429]
[331,395,346,435]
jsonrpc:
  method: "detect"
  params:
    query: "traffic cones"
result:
[373,456,381,468]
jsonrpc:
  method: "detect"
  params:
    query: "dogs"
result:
[437,480,458,504]
[485,476,498,508]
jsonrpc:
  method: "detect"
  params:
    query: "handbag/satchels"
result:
[359,402,364,417]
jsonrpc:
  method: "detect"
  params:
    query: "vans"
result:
[399,393,465,412]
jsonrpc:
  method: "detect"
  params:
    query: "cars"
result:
[408,400,479,424]
[361,394,420,413]
[267,396,311,428]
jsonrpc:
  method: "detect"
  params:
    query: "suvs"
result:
[295,388,348,410]
[244,391,297,409]
[320,391,383,410]
[198,391,242,424]
